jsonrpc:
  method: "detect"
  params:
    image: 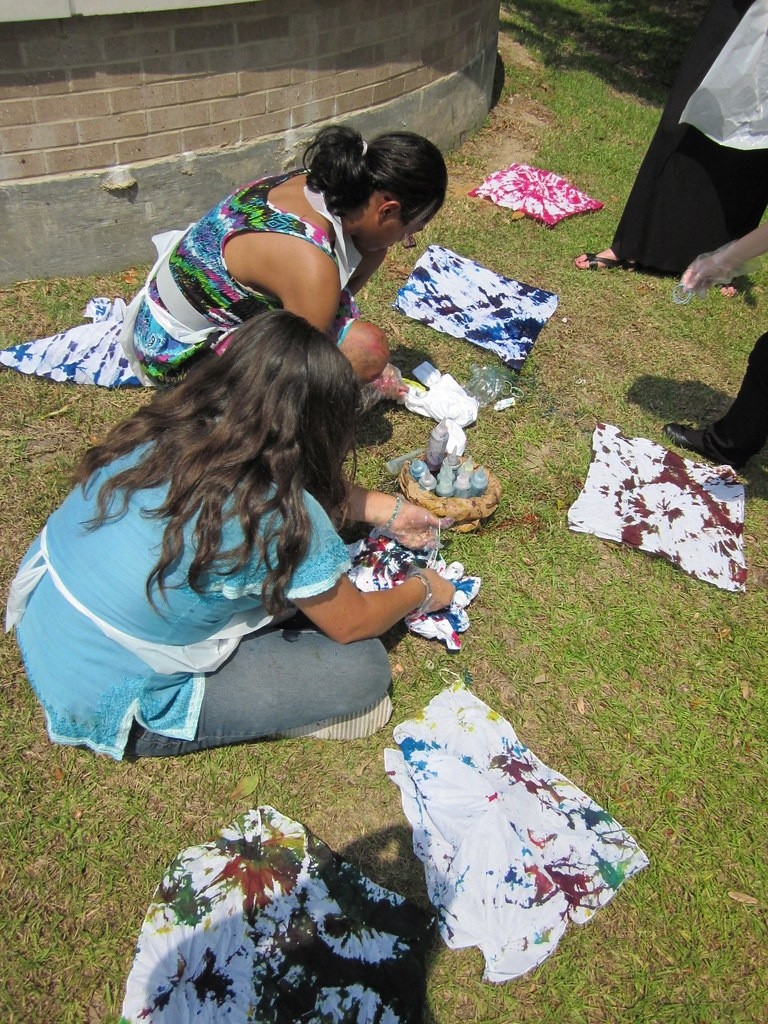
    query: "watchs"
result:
[414,573,433,612]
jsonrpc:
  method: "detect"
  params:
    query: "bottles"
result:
[383,417,488,499]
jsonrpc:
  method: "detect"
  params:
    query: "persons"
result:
[4,311,455,762]
[573,0,768,296]
[663,221,768,468]
[131,125,448,390]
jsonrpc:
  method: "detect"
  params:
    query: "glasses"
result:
[383,195,417,248]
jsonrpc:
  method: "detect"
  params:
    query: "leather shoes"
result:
[665,423,704,455]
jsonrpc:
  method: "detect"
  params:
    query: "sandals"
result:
[578,253,638,272]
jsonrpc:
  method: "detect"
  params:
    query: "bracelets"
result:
[382,496,401,530]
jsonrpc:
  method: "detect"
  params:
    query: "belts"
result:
[156,242,221,332]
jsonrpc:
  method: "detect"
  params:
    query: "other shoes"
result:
[267,690,393,741]
